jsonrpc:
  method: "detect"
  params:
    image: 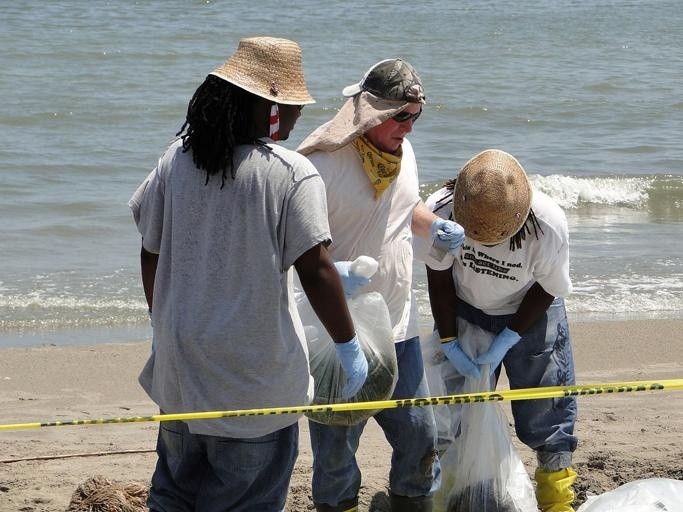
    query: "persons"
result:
[411,149,578,512]
[291,55,468,512]
[124,35,368,511]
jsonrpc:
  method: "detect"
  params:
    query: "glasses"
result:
[391,111,421,123]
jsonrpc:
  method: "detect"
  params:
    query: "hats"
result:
[208,36,317,106]
[452,149,532,245]
[343,58,425,103]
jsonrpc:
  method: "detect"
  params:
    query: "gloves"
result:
[441,339,480,379]
[333,332,368,398]
[333,261,370,299]
[430,218,464,253]
[476,327,522,372]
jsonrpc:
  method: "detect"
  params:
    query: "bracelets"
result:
[438,336,457,343]
